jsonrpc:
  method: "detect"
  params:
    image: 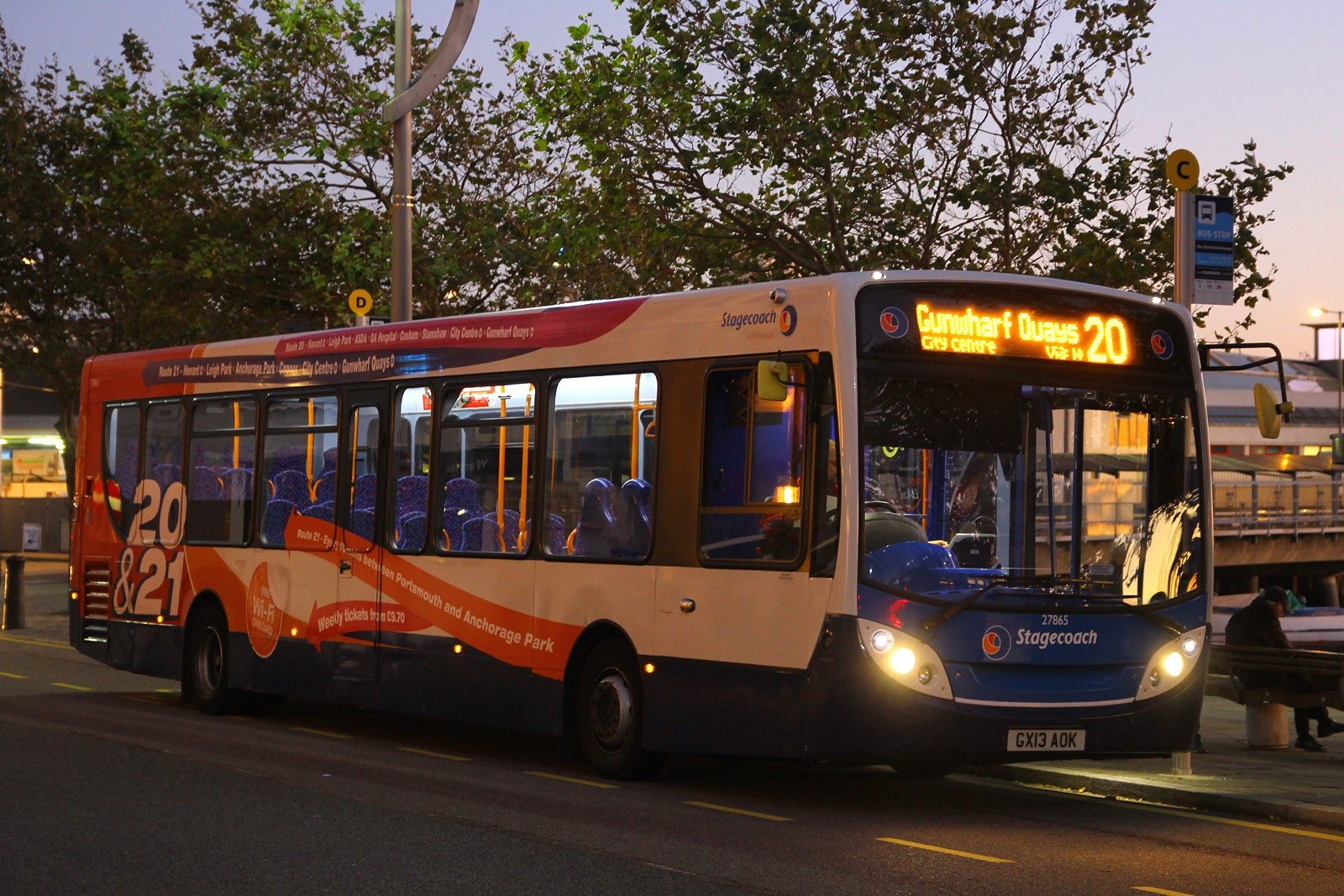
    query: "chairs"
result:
[138,460,753,560]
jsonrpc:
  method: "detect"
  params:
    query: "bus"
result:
[69,266,1295,785]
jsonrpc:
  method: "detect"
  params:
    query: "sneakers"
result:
[1318,720,1344,737]
[1295,735,1327,751]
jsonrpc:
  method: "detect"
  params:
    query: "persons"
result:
[1225,585,1344,752]
[760,438,889,550]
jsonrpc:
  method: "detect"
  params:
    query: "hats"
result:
[1261,585,1297,614]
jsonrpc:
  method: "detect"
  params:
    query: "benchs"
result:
[1205,643,1344,750]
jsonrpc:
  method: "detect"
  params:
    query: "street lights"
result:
[1309,308,1344,432]
